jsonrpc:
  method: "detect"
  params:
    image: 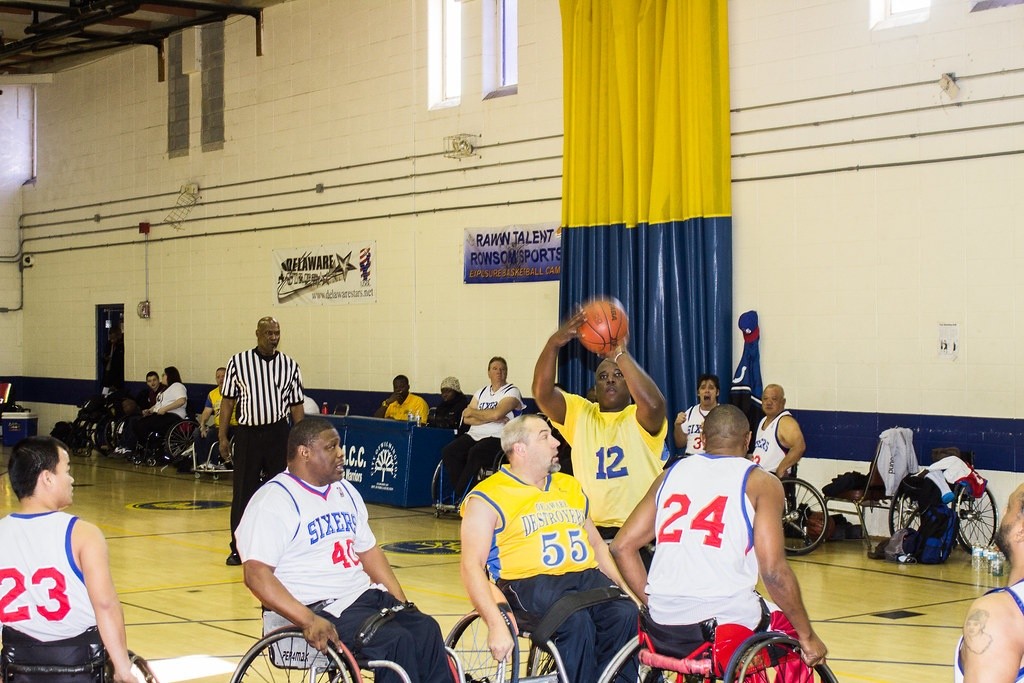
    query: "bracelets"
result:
[614,347,628,361]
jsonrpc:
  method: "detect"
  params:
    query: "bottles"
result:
[322,402,329,414]
[408,409,414,421]
[416,410,421,427]
[970,540,1011,577]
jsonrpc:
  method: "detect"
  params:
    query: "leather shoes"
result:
[226,551,241,565]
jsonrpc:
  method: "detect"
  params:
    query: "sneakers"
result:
[192,463,216,473]
[212,459,233,472]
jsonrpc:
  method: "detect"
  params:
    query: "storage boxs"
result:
[2,413,37,445]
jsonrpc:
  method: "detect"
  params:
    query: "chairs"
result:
[822,429,915,550]
[333,404,350,415]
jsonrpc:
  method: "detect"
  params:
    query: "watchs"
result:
[382,401,388,408]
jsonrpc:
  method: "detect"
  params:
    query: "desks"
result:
[303,413,454,508]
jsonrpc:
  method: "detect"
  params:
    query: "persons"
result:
[532,309,668,567]
[752,383,806,478]
[219,316,304,566]
[955,484,1024,683]
[608,403,827,683]
[434,356,527,505]
[116,366,187,452]
[460,414,664,683]
[375,375,429,423]
[0,437,138,683]
[235,418,453,683]
[675,374,720,455]
[196,367,237,470]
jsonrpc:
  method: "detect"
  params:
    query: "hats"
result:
[738,311,760,342]
[440,377,460,392]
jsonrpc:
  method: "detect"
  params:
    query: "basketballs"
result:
[808,512,836,539]
[576,301,629,355]
[123,399,137,415]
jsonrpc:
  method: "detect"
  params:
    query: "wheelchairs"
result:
[0,633,162,683]
[443,600,573,683]
[760,459,830,557]
[228,602,465,683]
[886,449,998,556]
[429,448,509,522]
[593,605,840,683]
[69,400,237,481]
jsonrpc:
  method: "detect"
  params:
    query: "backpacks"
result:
[882,505,960,566]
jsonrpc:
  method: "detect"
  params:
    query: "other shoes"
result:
[433,497,457,509]
